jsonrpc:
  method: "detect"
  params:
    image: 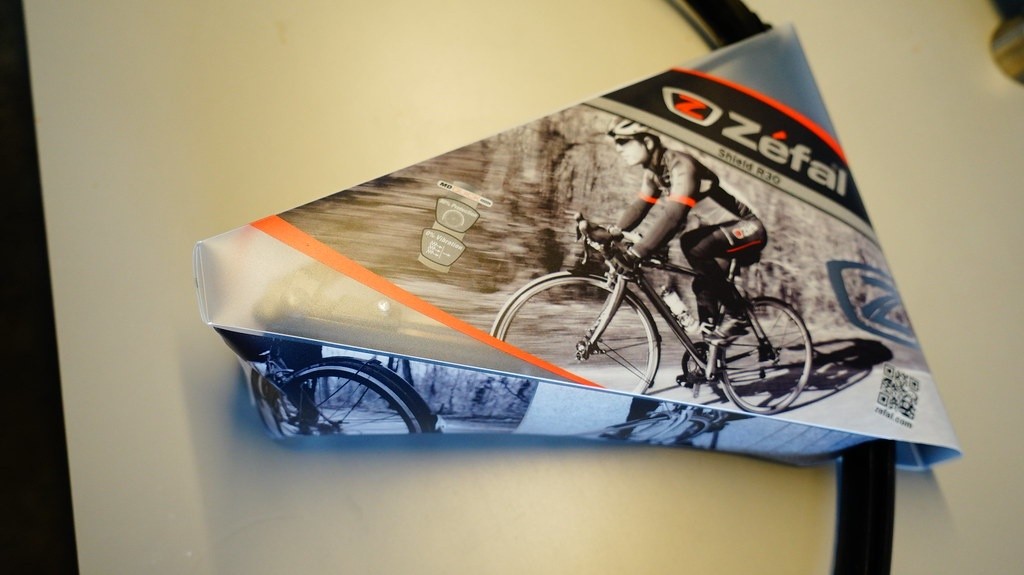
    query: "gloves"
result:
[610,253,637,275]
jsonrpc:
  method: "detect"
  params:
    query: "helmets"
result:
[608,119,660,139]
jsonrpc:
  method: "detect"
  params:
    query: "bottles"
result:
[660,286,699,335]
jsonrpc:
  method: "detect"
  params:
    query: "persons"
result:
[579,116,767,386]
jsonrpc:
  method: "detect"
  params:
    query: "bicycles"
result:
[247,340,444,434]
[486,207,813,417]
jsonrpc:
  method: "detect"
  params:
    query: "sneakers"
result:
[676,367,720,387]
[706,319,752,341]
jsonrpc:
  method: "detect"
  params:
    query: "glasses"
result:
[616,138,630,145]
